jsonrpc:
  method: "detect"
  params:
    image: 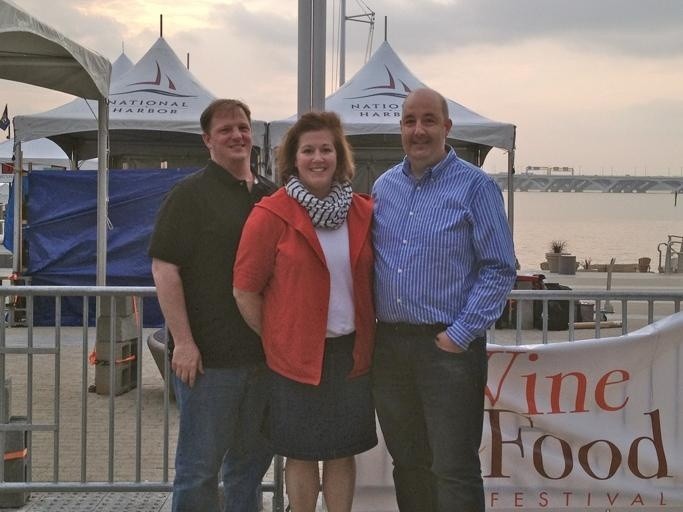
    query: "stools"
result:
[558,256,578,274]
[0,273,34,329]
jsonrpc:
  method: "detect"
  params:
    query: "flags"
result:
[0,104,10,131]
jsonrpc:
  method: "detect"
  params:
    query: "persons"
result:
[146,100,283,512]
[231,110,381,512]
[370,87,517,512]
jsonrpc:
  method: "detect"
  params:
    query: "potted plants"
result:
[546,241,570,271]
